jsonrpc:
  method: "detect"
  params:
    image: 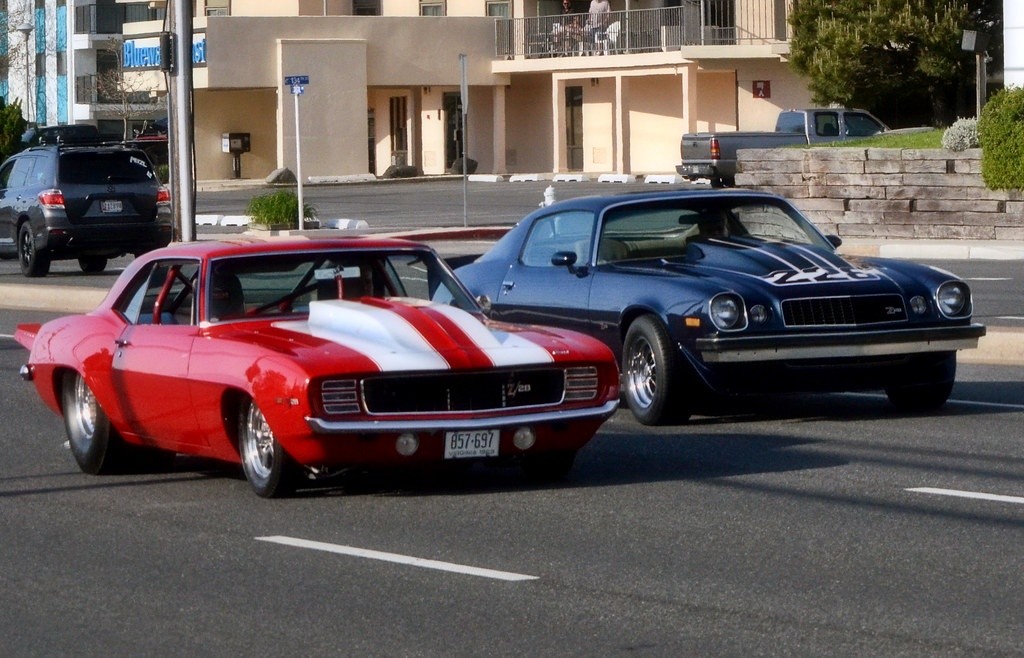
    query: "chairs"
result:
[576,238,633,267]
[595,20,621,55]
[191,271,246,321]
[679,220,751,245]
[314,259,373,301]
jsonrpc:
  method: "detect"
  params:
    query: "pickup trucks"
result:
[675,107,892,188]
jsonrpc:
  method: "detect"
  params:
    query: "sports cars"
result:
[429,187,987,428]
[8,226,624,500]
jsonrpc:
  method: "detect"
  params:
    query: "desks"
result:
[530,33,559,58]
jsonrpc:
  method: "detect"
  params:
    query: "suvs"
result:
[0,125,178,278]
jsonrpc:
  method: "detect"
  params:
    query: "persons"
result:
[696,208,732,244]
[560,0,574,56]
[588,0,612,55]
